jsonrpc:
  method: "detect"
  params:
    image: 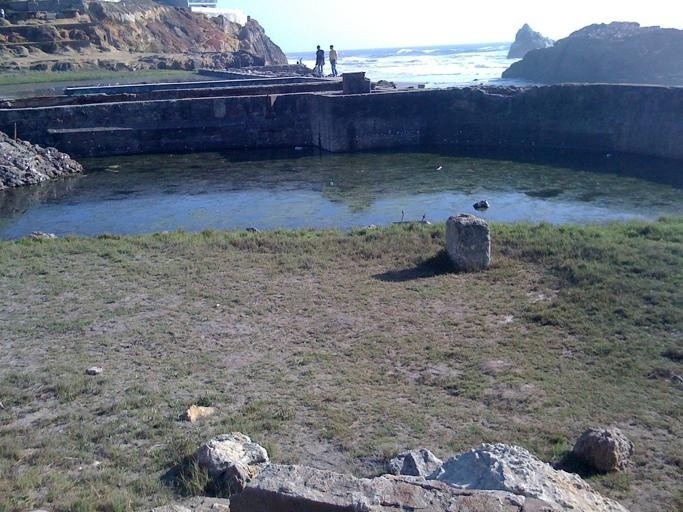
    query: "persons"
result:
[329,45,338,77]
[316,45,325,77]
